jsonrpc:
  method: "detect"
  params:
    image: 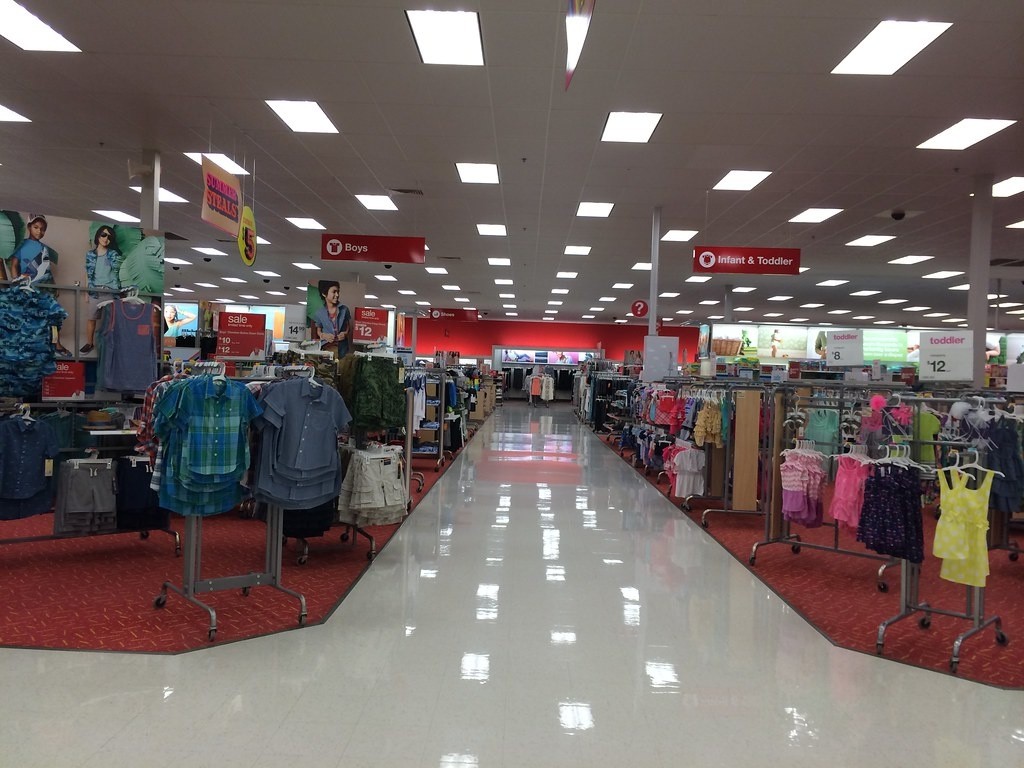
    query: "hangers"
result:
[354,342,398,364]
[292,339,334,360]
[120,443,152,467]
[172,359,227,383]
[403,367,426,381]
[597,369,1024,481]
[96,285,161,313]
[247,365,322,387]
[9,402,36,424]
[349,441,403,464]
[443,411,461,423]
[67,448,113,471]
[12,274,34,292]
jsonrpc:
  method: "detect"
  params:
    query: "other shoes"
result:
[80,343,94,354]
[52,343,71,357]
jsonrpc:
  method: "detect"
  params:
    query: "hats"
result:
[27,212,47,227]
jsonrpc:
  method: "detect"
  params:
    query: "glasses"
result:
[100,231,113,240]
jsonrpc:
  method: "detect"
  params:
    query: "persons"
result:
[815,330,827,359]
[655,316,663,336]
[435,349,461,367]
[503,349,532,362]
[77,224,126,355]
[11,213,74,358]
[269,348,303,366]
[555,351,574,365]
[202,302,214,333]
[905,344,920,362]
[313,280,352,359]
[770,328,781,358]
[249,347,265,361]
[396,312,406,349]
[161,305,196,347]
[625,349,643,365]
[983,334,1001,369]
[376,334,388,344]
